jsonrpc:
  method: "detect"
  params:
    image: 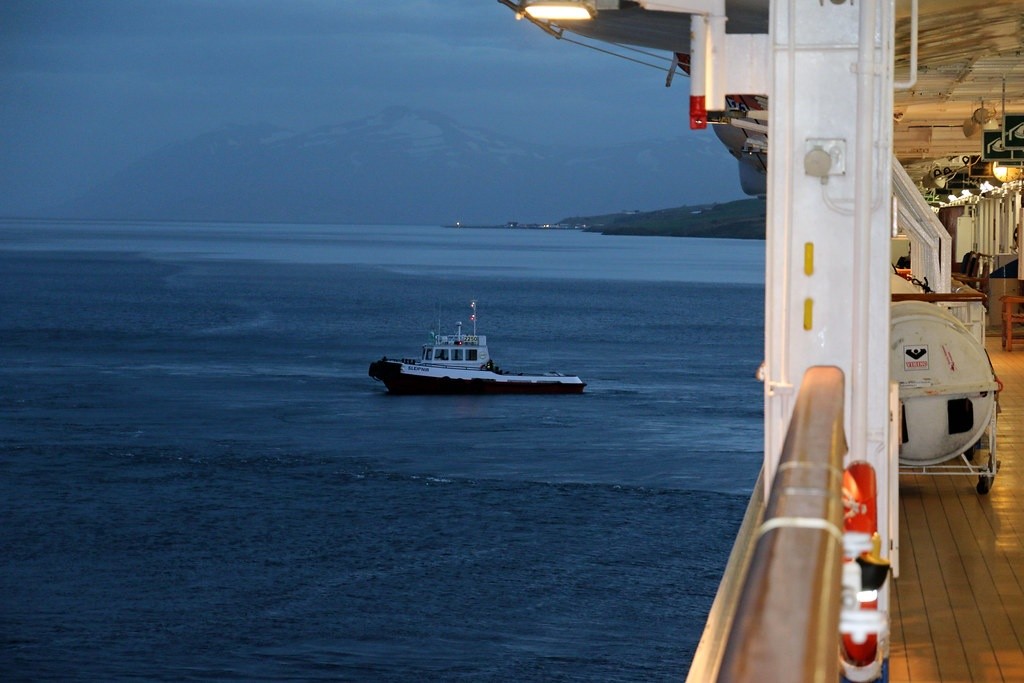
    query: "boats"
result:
[368,299,587,397]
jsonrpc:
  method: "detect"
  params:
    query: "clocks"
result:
[992,160,1022,184]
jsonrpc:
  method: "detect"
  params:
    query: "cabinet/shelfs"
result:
[998,296,1024,351]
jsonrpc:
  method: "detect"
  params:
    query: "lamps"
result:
[961,102,997,138]
[516,0,639,21]
